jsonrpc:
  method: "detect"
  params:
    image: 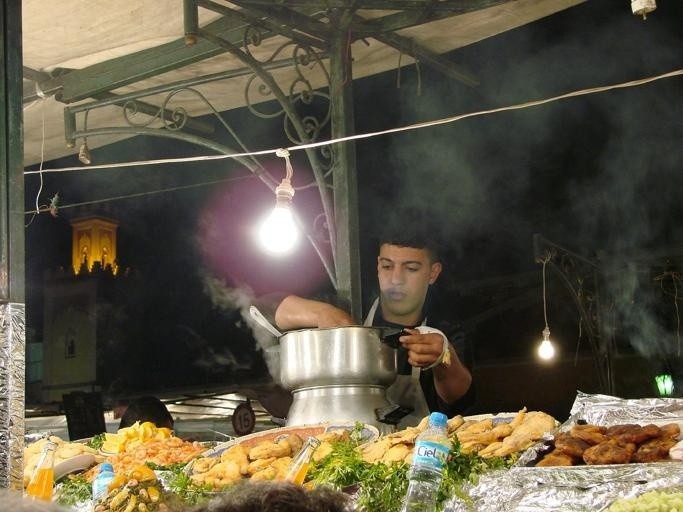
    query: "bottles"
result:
[400,409,451,511]
[92,462,114,495]
[29,439,58,505]
[274,435,321,486]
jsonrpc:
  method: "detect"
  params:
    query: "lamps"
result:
[538,261,555,359]
[256,149,305,265]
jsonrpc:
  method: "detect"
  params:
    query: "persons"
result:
[249,221,477,429]
[118,396,173,432]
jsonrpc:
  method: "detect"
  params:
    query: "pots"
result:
[268,326,404,427]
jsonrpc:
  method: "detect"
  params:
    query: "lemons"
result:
[102,421,171,452]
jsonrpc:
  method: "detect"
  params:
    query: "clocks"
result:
[80,245,109,262]
